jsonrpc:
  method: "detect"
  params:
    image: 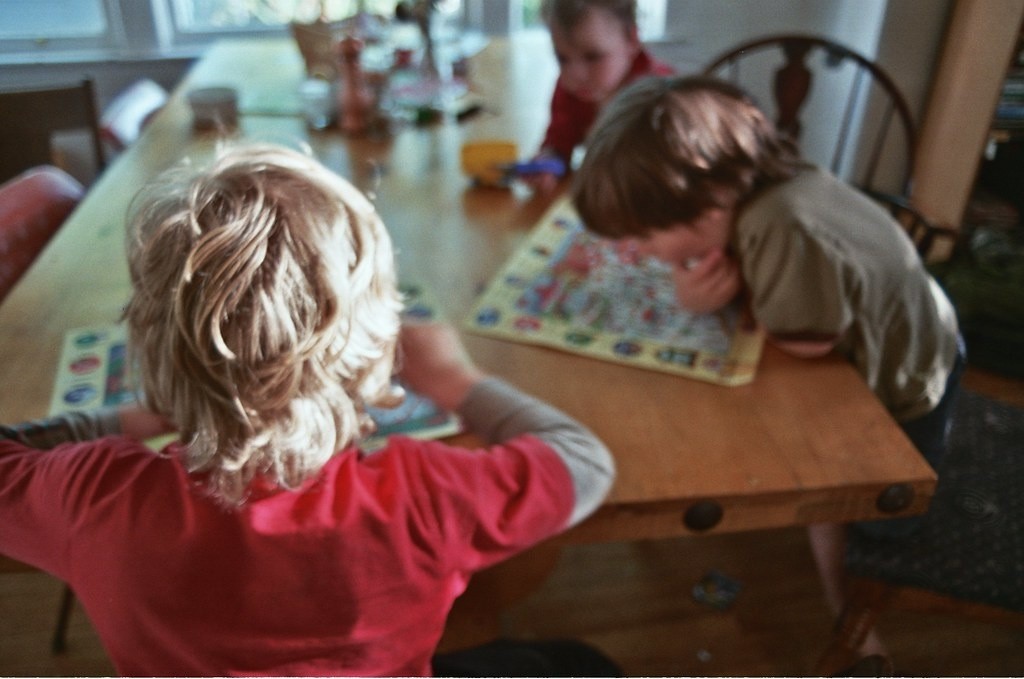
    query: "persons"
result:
[524,1,967,549]
[0,143,616,679]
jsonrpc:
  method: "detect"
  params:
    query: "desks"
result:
[1,35,937,676]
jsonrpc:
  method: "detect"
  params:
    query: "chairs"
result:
[0,165,85,301]
[702,35,974,278]
[95,78,169,151]
[1,77,106,186]
[807,389,1024,676]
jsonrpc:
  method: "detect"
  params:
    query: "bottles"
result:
[384,47,420,127]
[335,38,376,134]
[190,88,246,154]
